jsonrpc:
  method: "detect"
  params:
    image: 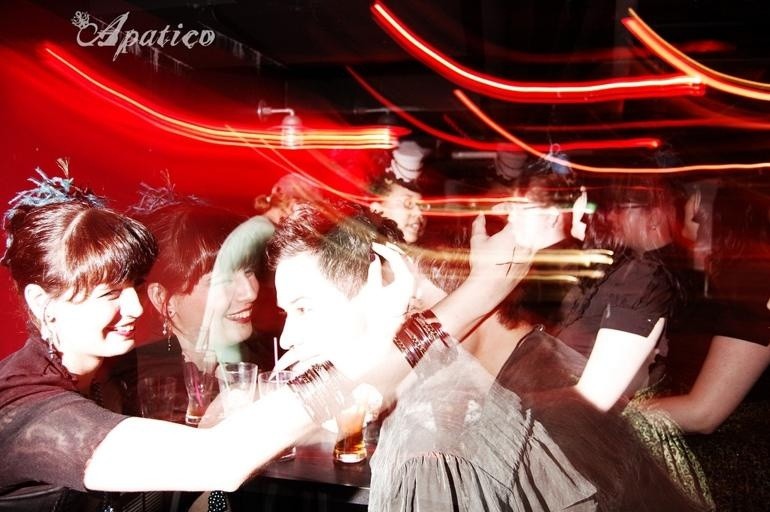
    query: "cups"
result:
[136,350,369,467]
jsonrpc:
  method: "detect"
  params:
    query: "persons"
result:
[0,165,770,512]
